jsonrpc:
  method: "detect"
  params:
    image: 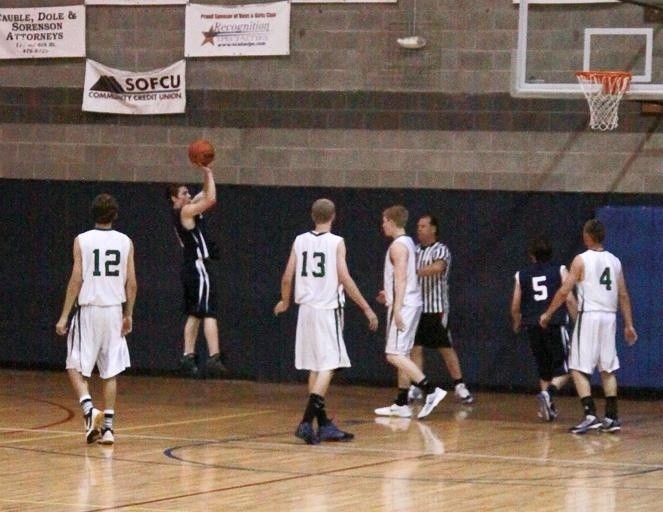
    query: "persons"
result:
[274,197,379,446]
[55,193,138,446]
[373,203,449,419]
[165,161,234,377]
[538,220,638,434]
[511,239,577,423]
[376,214,472,404]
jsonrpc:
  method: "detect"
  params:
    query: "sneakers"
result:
[200,350,237,379]
[454,382,475,404]
[96,425,118,447]
[293,420,323,446]
[535,389,555,424]
[82,407,107,446]
[599,416,622,433]
[372,402,414,419]
[316,420,354,444]
[567,413,605,435]
[177,352,206,382]
[415,386,449,419]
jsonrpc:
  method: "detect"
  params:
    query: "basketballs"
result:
[187,139,215,167]
